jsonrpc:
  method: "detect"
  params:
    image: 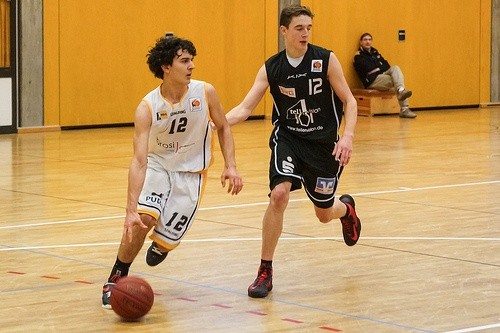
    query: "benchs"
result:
[351,88,396,117]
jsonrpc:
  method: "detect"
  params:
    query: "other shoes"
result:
[398,89,412,102]
[399,109,417,118]
[101,262,132,310]
[146,241,169,267]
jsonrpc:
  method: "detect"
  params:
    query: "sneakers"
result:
[247,265,275,298]
[339,194,362,247]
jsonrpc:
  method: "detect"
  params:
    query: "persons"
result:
[101,35,244,311]
[209,5,361,298]
[353,33,417,119]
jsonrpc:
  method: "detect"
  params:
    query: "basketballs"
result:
[110,278,154,318]
